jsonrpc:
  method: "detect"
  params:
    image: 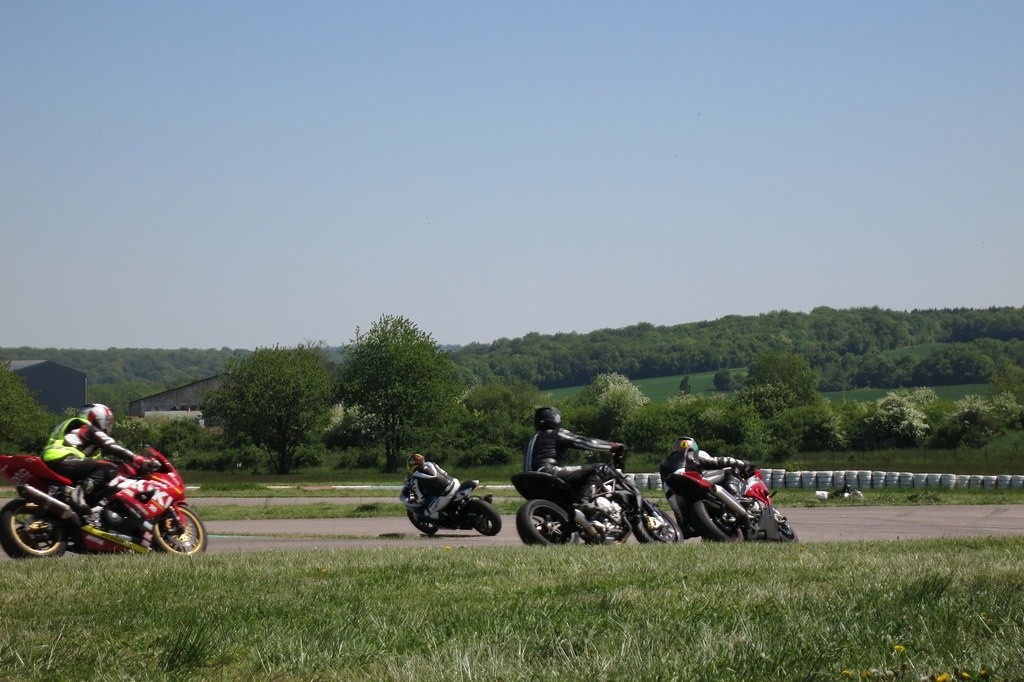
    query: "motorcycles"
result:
[0,443,208,560]
[665,466,798,544]
[511,446,684,547]
[399,476,502,536]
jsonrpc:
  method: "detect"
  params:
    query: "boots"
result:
[729,484,750,503]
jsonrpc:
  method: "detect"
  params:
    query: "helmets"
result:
[407,453,425,473]
[78,402,113,435]
[534,406,563,432]
[674,435,698,452]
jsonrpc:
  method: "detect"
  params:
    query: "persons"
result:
[524,403,628,501]
[658,435,755,503]
[39,402,162,515]
[400,452,482,528]
